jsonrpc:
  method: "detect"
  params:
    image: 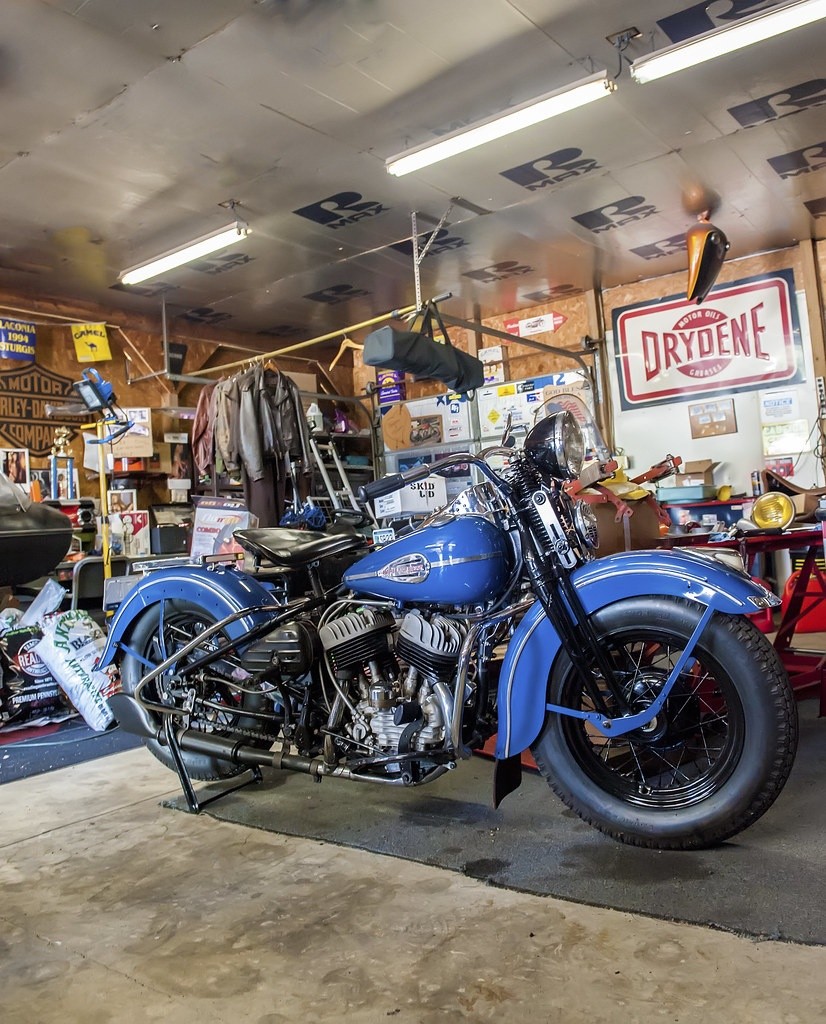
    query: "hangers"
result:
[221,354,278,378]
[328,328,364,372]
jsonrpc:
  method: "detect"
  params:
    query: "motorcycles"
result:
[92,415,798,851]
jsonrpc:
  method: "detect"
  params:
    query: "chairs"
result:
[71,555,131,634]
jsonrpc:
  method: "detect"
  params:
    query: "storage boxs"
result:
[281,371,318,414]
[383,442,484,502]
[675,458,721,487]
[475,365,602,440]
[148,501,193,554]
[190,495,259,571]
[656,485,717,502]
[374,472,448,519]
[377,369,406,404]
[477,344,511,385]
[791,493,819,522]
[377,390,481,454]
[96,509,150,556]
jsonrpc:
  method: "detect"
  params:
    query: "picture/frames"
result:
[0,448,31,494]
[31,468,52,500]
[57,468,81,499]
[107,489,137,515]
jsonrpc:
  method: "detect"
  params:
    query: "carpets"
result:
[156,692,826,949]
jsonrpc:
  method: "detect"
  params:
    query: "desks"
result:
[660,530,826,733]
[54,553,190,569]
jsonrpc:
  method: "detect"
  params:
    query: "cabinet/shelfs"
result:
[309,431,374,496]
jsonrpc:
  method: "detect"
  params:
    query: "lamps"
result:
[385,68,619,178]
[117,220,253,286]
[628,0,826,85]
